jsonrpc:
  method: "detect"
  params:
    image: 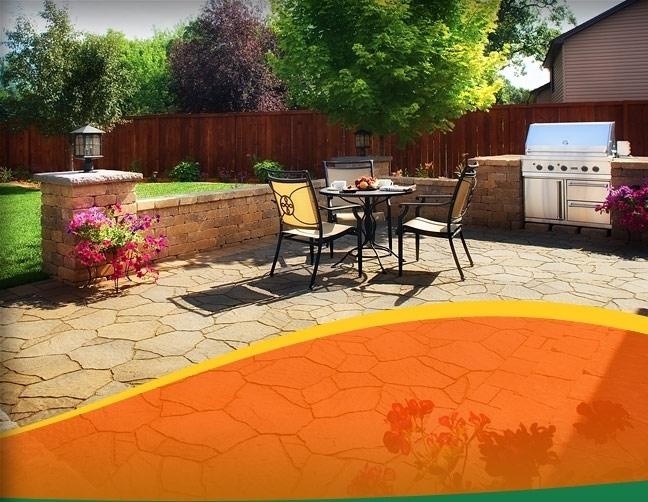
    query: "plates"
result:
[326,187,359,193]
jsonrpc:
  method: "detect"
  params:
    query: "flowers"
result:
[64,202,168,285]
[595,184,648,233]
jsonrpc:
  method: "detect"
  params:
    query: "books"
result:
[341,186,360,192]
[379,184,417,192]
[339,189,356,194]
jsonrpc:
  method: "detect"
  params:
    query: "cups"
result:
[378,179,394,187]
[331,180,347,189]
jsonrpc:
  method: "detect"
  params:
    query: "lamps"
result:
[354,130,372,156]
[69,122,107,172]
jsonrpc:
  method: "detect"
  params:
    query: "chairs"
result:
[261,160,479,290]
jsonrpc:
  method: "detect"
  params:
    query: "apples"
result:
[355,177,376,188]
